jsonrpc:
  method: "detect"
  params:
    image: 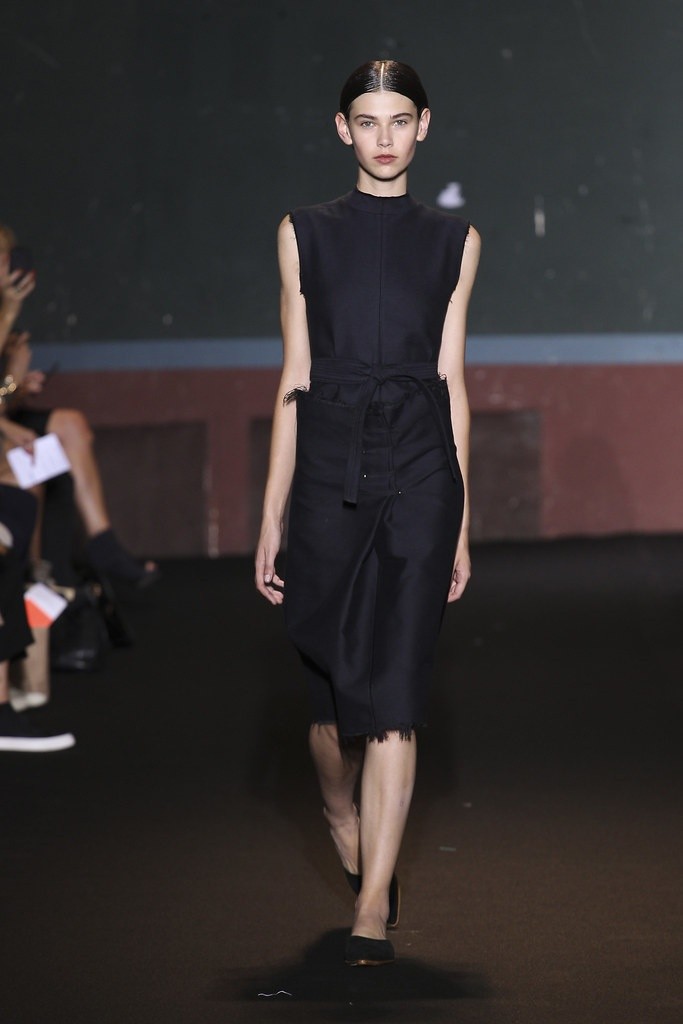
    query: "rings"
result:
[14,285,21,292]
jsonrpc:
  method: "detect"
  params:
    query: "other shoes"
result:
[344,856,401,929]
[343,934,395,966]
[94,585,134,649]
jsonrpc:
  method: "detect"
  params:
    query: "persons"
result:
[255,61,482,966]
[0,227,156,754]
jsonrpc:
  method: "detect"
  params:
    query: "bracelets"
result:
[0,380,17,402]
